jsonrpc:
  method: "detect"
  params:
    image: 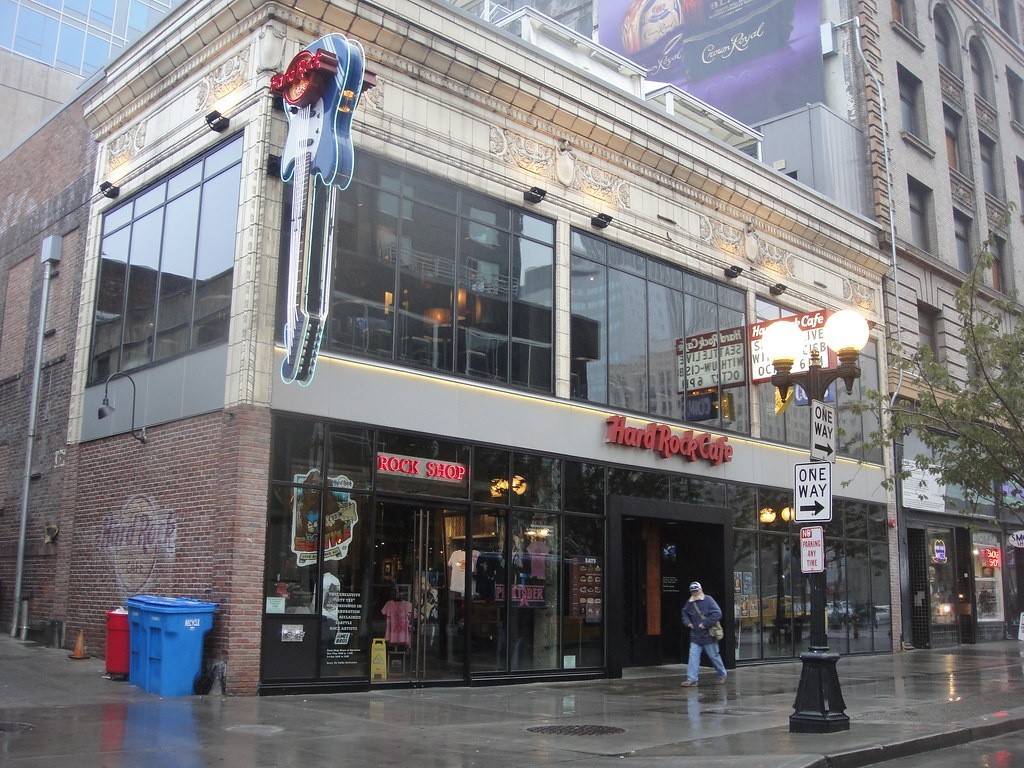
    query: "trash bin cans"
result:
[127,596,219,697]
[105,610,129,679]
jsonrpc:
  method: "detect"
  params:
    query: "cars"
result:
[740,593,891,632]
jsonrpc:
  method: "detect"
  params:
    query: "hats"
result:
[689,582,702,592]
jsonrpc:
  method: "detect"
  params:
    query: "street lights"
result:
[763,309,870,731]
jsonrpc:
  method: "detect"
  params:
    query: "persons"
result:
[682,582,727,686]
[853,599,878,630]
[414,571,438,622]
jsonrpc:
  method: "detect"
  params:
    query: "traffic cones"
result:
[68,629,90,660]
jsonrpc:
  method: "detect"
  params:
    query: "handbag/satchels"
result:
[700,615,724,640]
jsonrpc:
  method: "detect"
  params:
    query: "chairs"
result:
[398,316,432,366]
[457,335,490,380]
[362,304,394,352]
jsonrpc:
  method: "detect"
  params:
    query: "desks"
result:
[423,323,465,370]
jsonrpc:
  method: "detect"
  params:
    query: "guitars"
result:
[274,26,369,390]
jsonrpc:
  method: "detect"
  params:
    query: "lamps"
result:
[99,181,119,199]
[205,110,229,132]
[725,264,743,278]
[523,186,547,203]
[98,372,147,444]
[770,283,787,297]
[590,212,613,228]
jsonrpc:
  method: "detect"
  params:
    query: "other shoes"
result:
[719,675,727,684]
[681,680,698,687]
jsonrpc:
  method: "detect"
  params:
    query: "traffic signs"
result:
[809,398,839,464]
[793,460,833,523]
